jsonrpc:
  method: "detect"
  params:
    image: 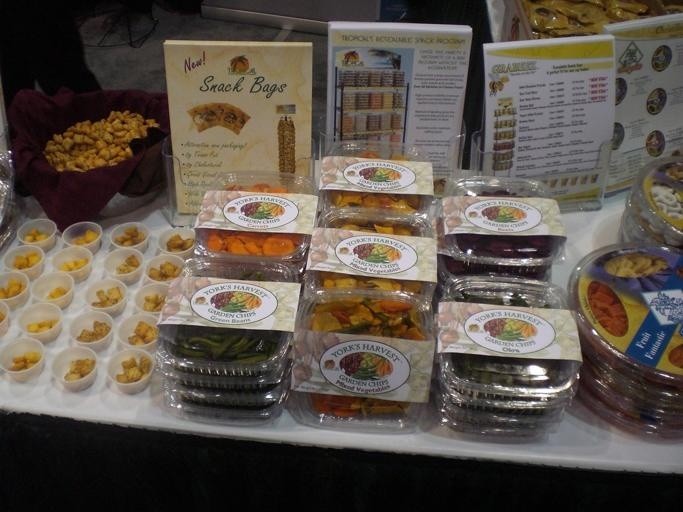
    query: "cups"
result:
[105,347,155,395]
[52,347,98,392]
[1,216,195,352]
[0,337,46,384]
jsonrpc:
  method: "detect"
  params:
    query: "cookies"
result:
[668,345,682,368]
[604,251,669,277]
[588,281,629,336]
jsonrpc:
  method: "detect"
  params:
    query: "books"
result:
[603,11,683,193]
[483,38,616,205]
[326,22,472,179]
[165,40,313,215]
